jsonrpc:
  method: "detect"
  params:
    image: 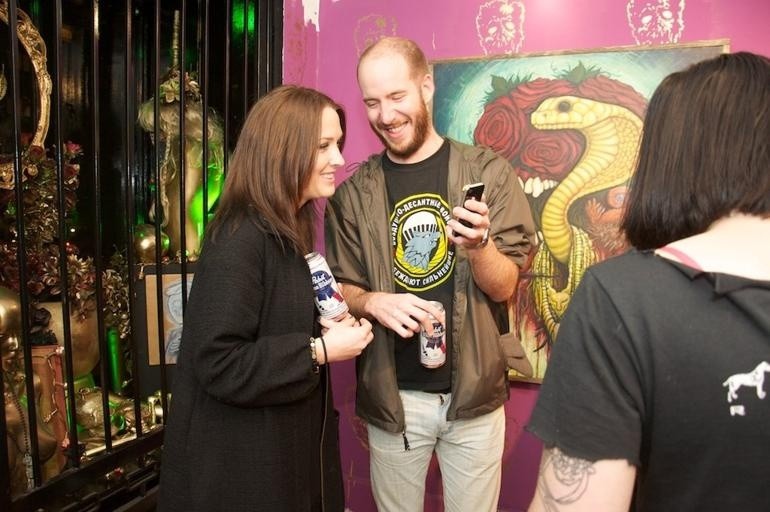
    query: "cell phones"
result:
[450,182,485,245]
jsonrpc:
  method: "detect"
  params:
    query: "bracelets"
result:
[320,335,328,365]
[308,336,320,370]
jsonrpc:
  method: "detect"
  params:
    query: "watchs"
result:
[467,227,491,248]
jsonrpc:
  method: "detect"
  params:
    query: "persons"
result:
[528,53,770,511]
[324,34,535,511]
[158,84,376,510]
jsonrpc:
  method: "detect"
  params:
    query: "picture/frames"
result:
[428,36,730,389]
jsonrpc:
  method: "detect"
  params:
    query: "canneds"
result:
[421,301,447,368]
[304,251,349,319]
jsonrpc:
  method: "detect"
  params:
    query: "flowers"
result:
[469,57,649,185]
